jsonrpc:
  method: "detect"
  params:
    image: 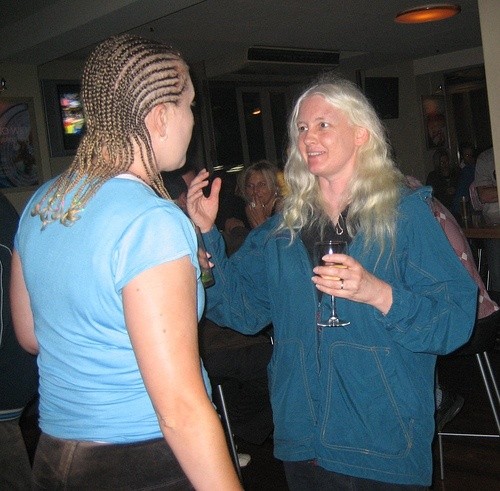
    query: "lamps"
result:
[395,3,461,24]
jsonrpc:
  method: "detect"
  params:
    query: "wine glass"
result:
[314,241,350,328]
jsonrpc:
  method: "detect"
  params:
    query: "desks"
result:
[453,212,500,290]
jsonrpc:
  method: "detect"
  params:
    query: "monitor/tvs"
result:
[41,78,87,158]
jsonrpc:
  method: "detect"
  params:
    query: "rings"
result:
[341,280,343,290]
[251,203,255,207]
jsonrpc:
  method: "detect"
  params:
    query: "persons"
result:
[186,78,479,491]
[10,34,246,491]
[0,160,500,491]
[423,144,500,231]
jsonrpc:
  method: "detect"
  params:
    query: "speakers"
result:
[365,77,399,119]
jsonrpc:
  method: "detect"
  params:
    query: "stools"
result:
[435,336,500,480]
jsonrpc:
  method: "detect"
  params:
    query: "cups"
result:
[194,226,215,288]
[453,194,474,229]
